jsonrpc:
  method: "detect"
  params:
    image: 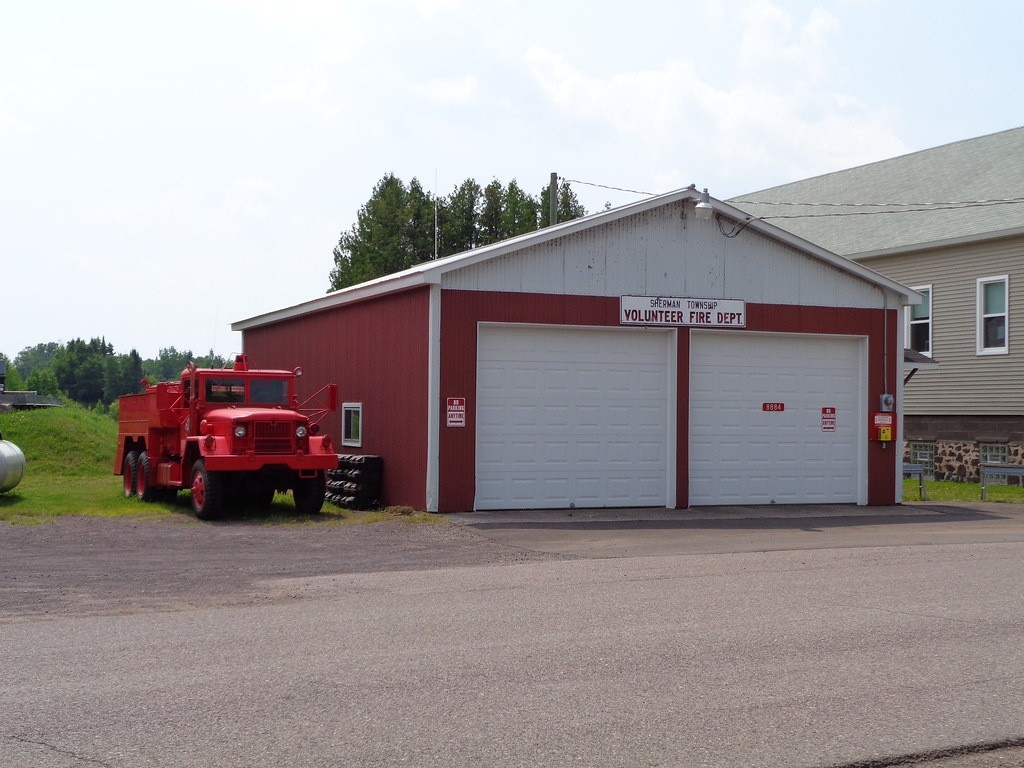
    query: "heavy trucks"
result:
[113,350,340,521]
